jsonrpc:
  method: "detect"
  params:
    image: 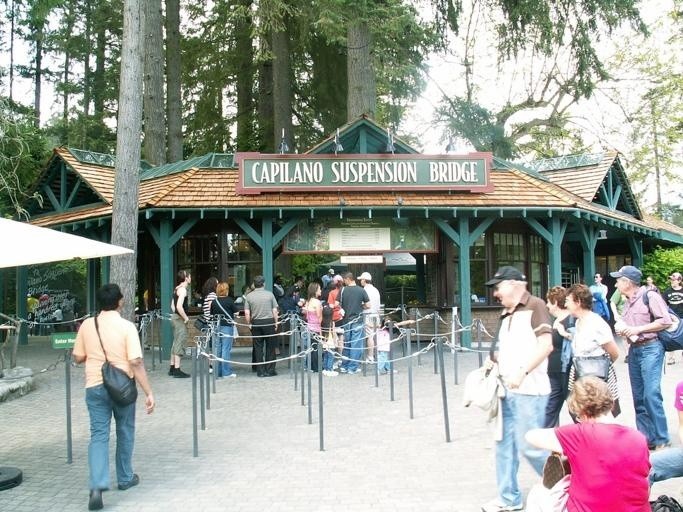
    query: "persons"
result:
[27,293,85,334]
[168,270,191,378]
[74,283,155,510]
[484,263,683,512]
[193,269,398,376]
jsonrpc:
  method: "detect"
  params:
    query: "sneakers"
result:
[88,490,103,510]
[321,360,361,377]
[481,498,523,512]
[167,370,191,378]
[223,374,236,378]
[118,472,139,490]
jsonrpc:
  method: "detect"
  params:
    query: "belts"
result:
[631,337,658,347]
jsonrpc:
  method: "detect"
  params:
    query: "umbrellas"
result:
[0,211,135,270]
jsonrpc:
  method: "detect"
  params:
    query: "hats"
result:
[485,266,527,286]
[357,272,372,280]
[609,265,642,283]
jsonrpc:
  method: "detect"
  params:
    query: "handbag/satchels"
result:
[649,495,682,512]
[102,361,137,406]
[193,317,206,330]
[462,366,498,422]
[653,309,683,351]
[572,357,609,382]
[542,455,566,489]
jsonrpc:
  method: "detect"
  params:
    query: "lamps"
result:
[381,127,396,155]
[329,127,344,157]
[277,127,290,155]
